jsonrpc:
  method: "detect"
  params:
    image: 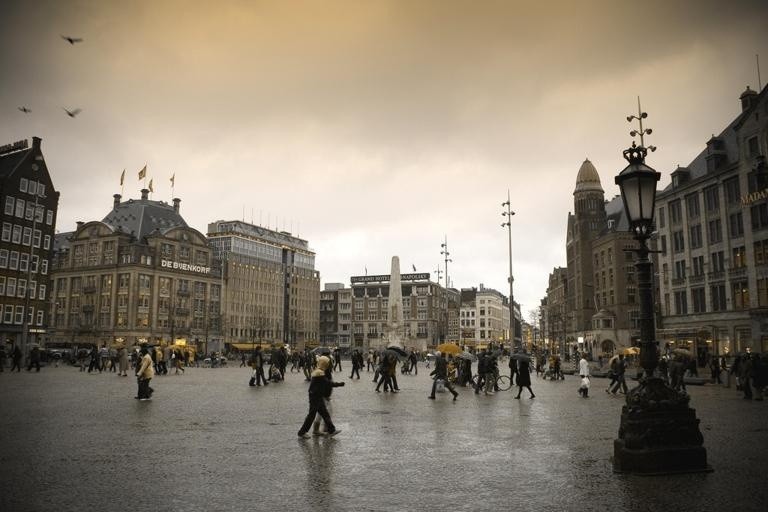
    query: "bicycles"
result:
[471,373,512,391]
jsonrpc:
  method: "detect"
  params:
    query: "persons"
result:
[577,352,591,398]
[312,356,334,436]
[605,356,624,393]
[343,343,421,393]
[297,355,342,438]
[135,348,156,400]
[133,348,154,399]
[249,343,343,386]
[609,353,630,394]
[0,339,247,376]
[622,346,768,402]
[421,343,565,401]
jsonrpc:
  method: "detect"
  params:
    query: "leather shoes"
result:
[330,429,342,437]
[391,390,396,393]
[384,389,389,392]
[376,389,381,393]
[395,388,400,390]
[428,397,435,399]
[453,392,459,401]
[529,394,535,399]
[487,391,494,396]
[514,396,520,399]
[298,433,311,439]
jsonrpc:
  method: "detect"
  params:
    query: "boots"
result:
[605,384,611,394]
[313,421,324,436]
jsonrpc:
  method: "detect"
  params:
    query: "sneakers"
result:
[257,377,285,386]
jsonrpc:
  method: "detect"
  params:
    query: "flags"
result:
[120,169,125,186]
[169,175,174,186]
[138,165,146,179]
[149,179,154,193]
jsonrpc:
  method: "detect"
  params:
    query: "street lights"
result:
[613,142,705,448]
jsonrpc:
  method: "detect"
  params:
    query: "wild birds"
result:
[17,106,33,114]
[60,33,83,46]
[63,105,82,118]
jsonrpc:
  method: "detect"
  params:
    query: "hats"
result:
[435,351,441,358]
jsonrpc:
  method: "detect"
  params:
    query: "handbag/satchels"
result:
[580,376,591,389]
[436,379,444,392]
